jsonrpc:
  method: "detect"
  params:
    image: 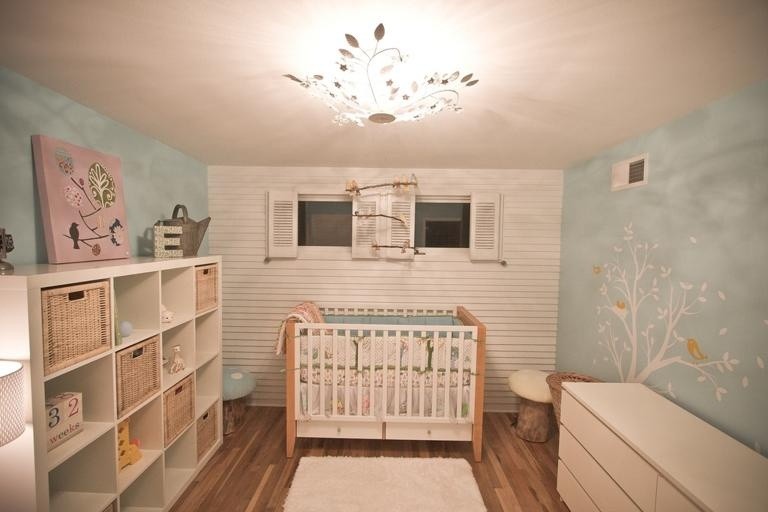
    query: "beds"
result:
[285,307,486,462]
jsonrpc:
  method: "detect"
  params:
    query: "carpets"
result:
[280,455,487,511]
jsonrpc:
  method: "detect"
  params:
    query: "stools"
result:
[223,366,255,435]
[510,368,558,444]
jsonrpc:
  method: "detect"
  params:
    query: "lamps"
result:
[282,22,480,131]
[0,359,25,446]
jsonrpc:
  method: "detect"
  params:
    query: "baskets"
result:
[546,371,605,429]
[164,373,195,447]
[196,265,218,316]
[197,405,217,461]
[39,280,114,375]
[115,335,162,420]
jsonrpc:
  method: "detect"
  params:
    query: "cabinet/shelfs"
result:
[556,382,766,511]
[0,256,224,511]
